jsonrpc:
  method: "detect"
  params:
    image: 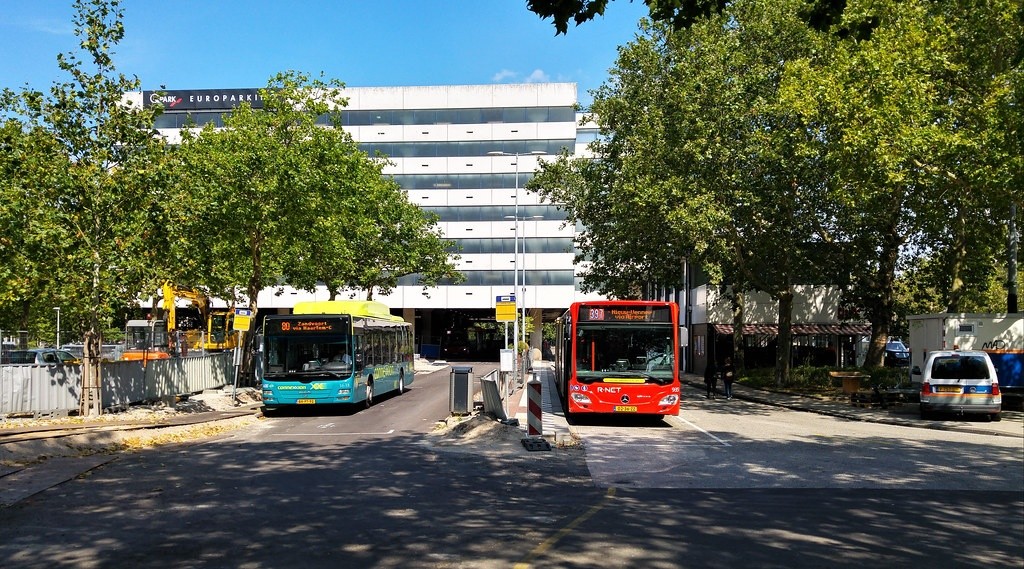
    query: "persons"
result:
[704,358,719,399]
[720,358,737,401]
[333,345,353,367]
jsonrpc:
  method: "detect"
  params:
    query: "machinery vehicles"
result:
[119,278,244,360]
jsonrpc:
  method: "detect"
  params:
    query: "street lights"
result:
[503,215,544,384]
[52,307,61,350]
[487,150,548,394]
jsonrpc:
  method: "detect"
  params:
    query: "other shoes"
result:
[708,396,711,399]
[713,396,716,399]
[727,397,732,401]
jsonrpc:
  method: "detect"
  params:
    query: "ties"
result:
[340,355,343,360]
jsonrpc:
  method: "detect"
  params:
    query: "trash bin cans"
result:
[449,366,474,417]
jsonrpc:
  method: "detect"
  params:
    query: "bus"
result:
[554,300,689,421]
[253,300,414,412]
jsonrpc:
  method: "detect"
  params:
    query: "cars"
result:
[884,342,911,367]
[911,350,1002,422]
[1,343,120,364]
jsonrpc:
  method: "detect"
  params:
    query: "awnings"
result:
[712,324,874,353]
